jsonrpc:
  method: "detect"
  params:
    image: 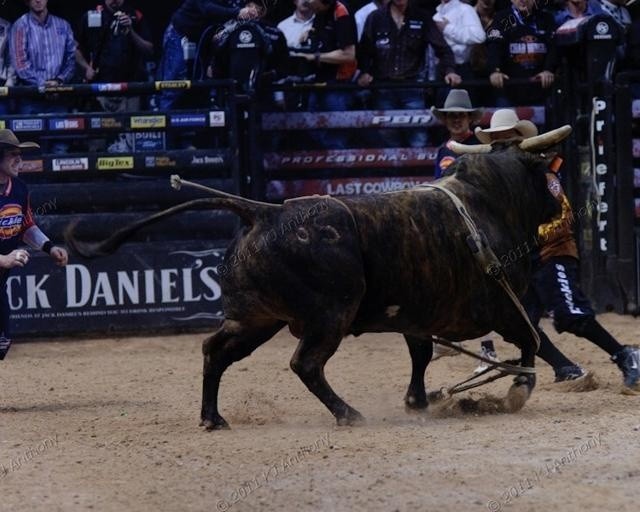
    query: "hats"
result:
[475,109,538,144]
[1,129,41,149]
[431,89,482,122]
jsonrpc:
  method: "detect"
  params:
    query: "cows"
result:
[67,123,572,432]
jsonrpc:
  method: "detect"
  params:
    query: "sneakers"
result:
[474,351,499,373]
[610,344,640,388]
[554,362,583,382]
[430,342,461,360]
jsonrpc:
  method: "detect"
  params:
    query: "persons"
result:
[483,128,640,391]
[1,129,68,362]
[426,111,499,372]
[0,0,639,142]
